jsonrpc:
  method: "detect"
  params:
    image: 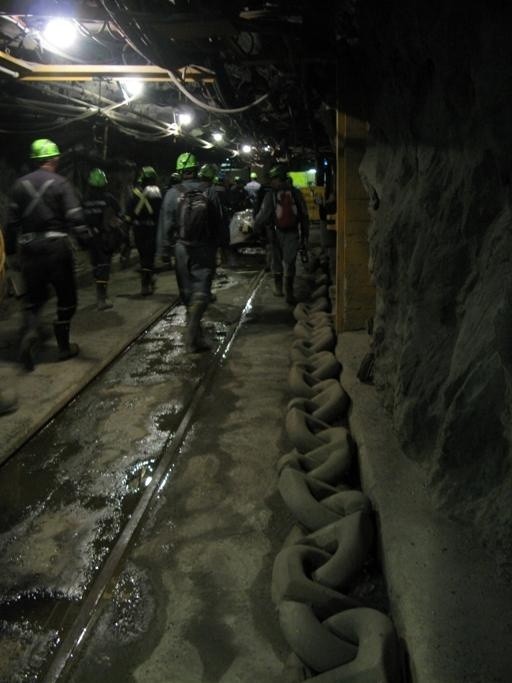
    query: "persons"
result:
[124,150,309,356]
[8,138,92,370]
[80,164,122,312]
[0,381,20,415]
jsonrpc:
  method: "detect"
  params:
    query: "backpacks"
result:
[173,182,222,251]
[272,187,302,232]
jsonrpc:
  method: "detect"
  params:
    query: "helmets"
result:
[269,166,284,178]
[28,138,63,160]
[199,163,219,183]
[137,166,160,184]
[175,152,200,171]
[249,172,259,179]
[87,167,109,188]
[234,176,241,182]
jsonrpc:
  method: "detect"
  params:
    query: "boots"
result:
[184,291,214,353]
[19,310,39,362]
[94,280,114,310]
[284,274,297,304]
[53,320,80,362]
[272,272,284,297]
[141,268,157,297]
